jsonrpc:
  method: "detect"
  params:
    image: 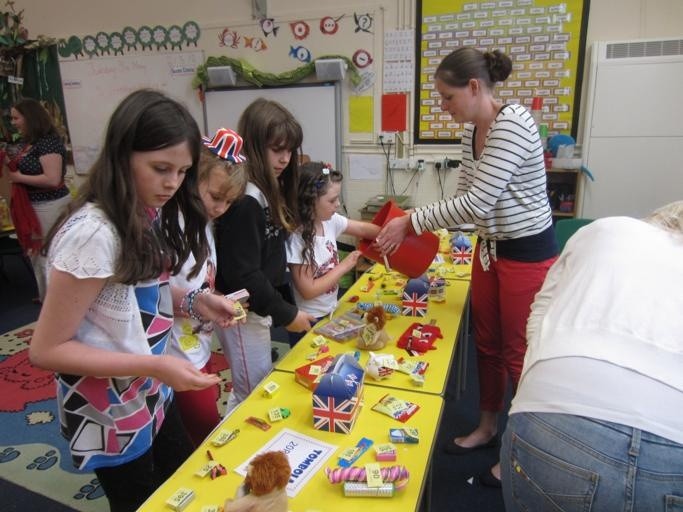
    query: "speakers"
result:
[207,66,236,88]
[315,59,346,80]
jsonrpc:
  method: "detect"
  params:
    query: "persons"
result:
[0,97,71,305]
[501,200,683,511]
[372,46,559,490]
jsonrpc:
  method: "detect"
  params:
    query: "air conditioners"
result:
[572,40,681,228]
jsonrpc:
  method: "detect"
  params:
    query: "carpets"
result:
[0,322,290,511]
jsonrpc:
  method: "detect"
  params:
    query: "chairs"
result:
[554,217,592,257]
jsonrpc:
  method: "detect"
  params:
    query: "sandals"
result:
[467,459,506,491]
[441,429,500,454]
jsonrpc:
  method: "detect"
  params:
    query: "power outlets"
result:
[434,158,456,170]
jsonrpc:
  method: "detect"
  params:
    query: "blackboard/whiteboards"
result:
[56,48,208,178]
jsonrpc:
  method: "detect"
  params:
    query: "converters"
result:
[447,161,459,167]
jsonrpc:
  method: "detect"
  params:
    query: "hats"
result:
[200,124,251,167]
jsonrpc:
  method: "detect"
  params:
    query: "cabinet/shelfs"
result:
[544,167,586,225]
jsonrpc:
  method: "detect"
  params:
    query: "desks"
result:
[136,229,478,511]
